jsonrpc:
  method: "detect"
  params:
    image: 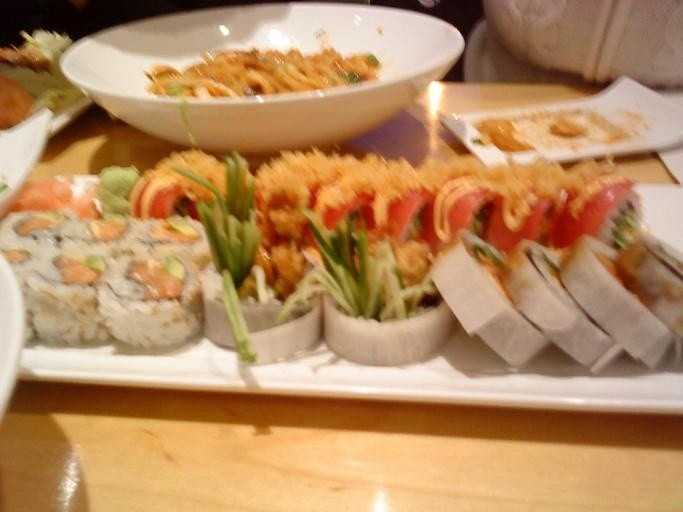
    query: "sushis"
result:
[0,148,681,375]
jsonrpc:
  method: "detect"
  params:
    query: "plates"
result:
[0,55,95,142]
[16,172,683,418]
[437,75,683,167]
[0,106,54,219]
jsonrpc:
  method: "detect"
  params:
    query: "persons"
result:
[460,0,682,90]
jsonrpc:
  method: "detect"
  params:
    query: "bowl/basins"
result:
[59,1,466,155]
